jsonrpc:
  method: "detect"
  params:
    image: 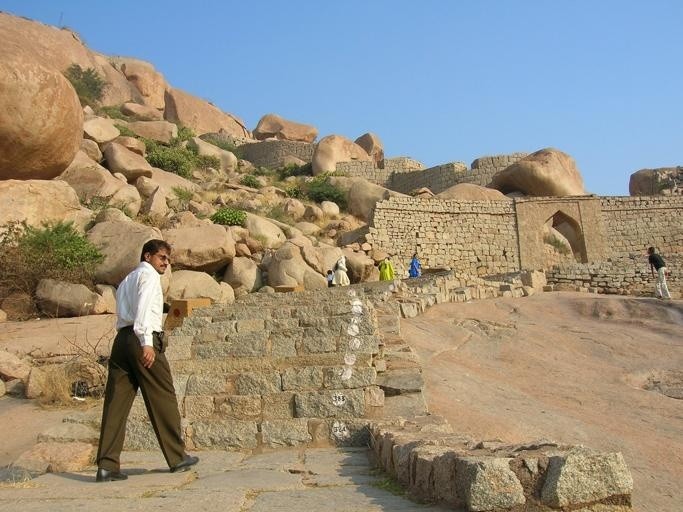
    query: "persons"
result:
[331,256,348,287]
[406,253,421,278]
[324,270,334,287]
[647,246,672,300]
[93,239,199,483]
[377,256,394,281]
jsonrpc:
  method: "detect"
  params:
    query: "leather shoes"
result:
[97,468,128,482]
[170,456,197,473]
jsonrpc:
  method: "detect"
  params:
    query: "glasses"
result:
[155,253,170,261]
